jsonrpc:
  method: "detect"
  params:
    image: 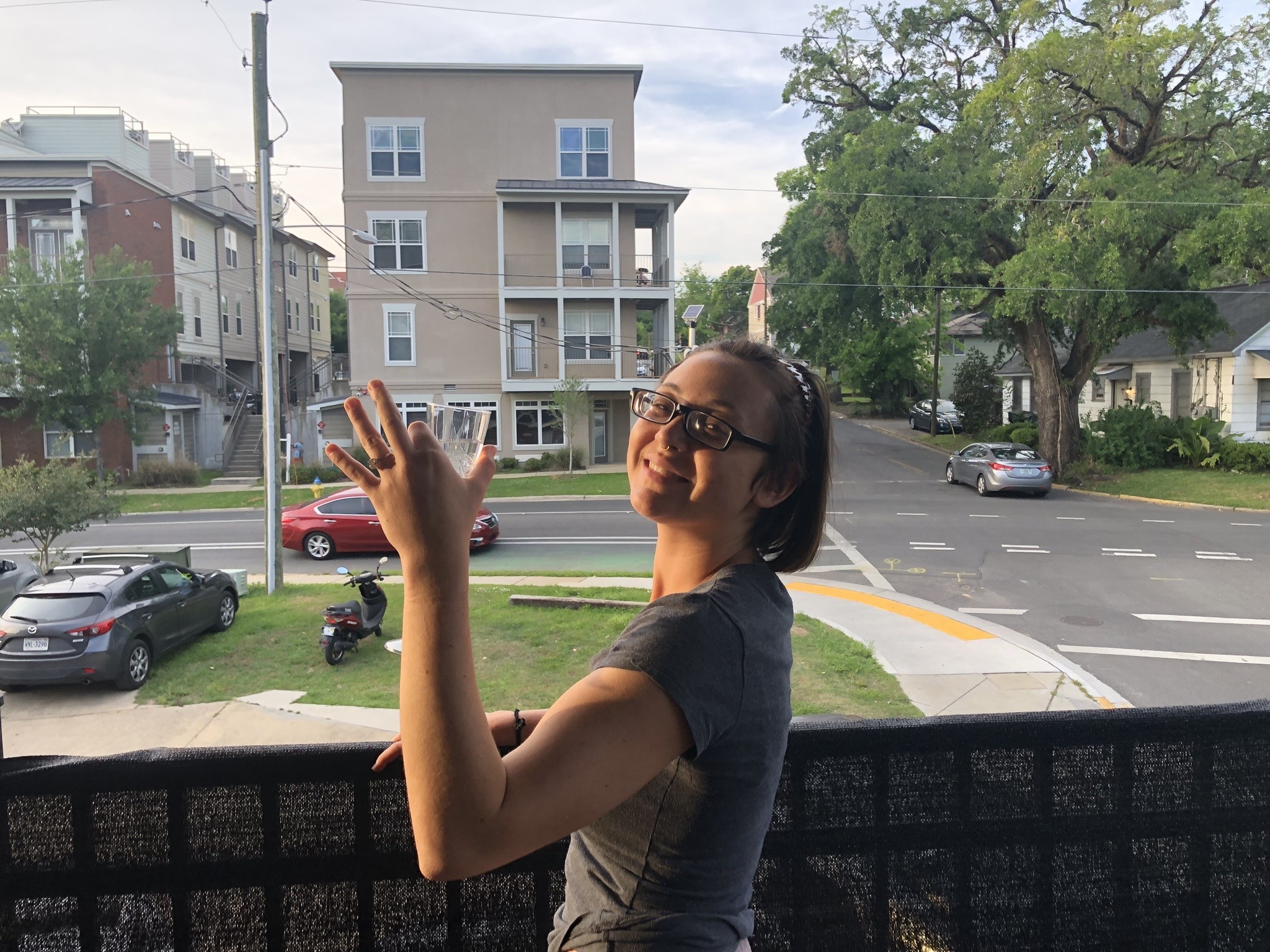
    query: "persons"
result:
[1124,387,1136,406]
[321,338,830,952]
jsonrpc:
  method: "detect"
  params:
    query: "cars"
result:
[0,557,239,693]
[637,349,649,359]
[945,442,1054,501]
[907,400,966,434]
[636,362,647,376]
[280,488,499,561]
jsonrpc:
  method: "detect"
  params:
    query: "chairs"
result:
[579,264,595,288]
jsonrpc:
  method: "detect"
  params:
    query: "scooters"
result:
[319,557,389,666]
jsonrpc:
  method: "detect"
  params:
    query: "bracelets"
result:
[514,709,526,744]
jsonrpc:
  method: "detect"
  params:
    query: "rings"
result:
[368,453,396,471]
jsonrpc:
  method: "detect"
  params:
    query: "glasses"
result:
[630,387,782,453]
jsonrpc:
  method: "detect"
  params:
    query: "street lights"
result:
[250,151,378,594]
[681,305,704,359]
